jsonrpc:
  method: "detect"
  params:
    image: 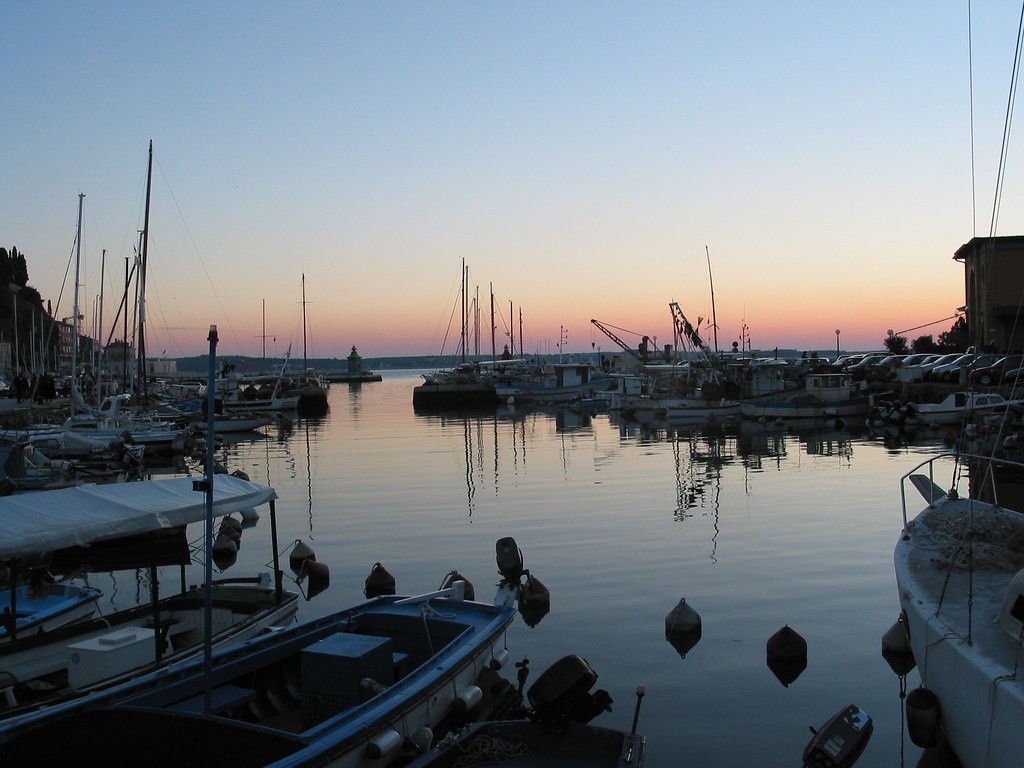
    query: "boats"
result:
[806,699,874,768]
[403,718,647,768]
[1,324,518,768]
[893,452,1023,768]
[1,472,301,726]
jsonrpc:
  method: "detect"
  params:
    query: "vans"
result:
[790,357,831,375]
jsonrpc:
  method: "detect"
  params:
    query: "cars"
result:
[931,354,981,382]
[876,354,909,368]
[755,359,789,367]
[841,356,888,375]
[950,354,1008,384]
[919,353,962,381]
[912,354,944,380]
[895,354,930,367]
[970,354,1024,386]
[831,356,864,374]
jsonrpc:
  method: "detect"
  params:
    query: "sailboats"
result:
[0,135,332,499]
[413,245,1024,438]
